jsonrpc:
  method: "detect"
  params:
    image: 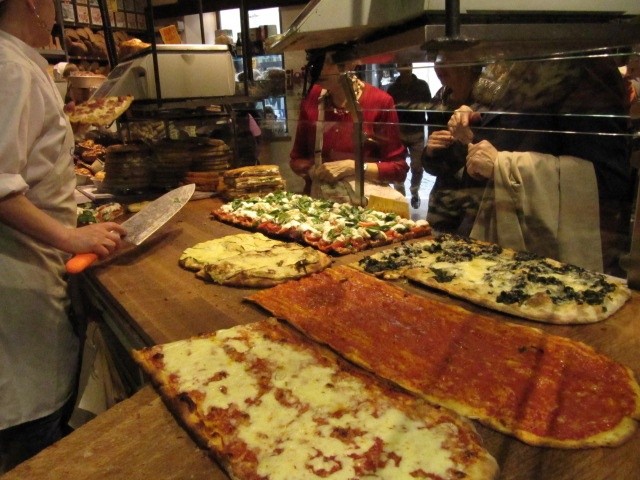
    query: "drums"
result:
[63,182,195,277]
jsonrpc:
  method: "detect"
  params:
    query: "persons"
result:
[421,62,491,234]
[289,55,410,204]
[0,1,127,473]
[53,63,81,80]
[447,58,630,274]
[232,101,262,167]
[386,62,431,209]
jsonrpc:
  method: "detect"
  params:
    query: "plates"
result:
[36,17,65,65]
[115,42,238,202]
[267,0,639,289]
[199,7,292,144]
[101,0,153,70]
[57,0,113,76]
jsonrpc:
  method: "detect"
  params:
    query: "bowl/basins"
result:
[325,76,363,116]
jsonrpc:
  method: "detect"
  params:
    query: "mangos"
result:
[68,94,135,125]
[178,231,335,288]
[209,190,431,255]
[349,233,632,325]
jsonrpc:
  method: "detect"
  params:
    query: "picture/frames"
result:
[0,188,640,479]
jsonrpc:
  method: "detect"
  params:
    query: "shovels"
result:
[243,265,639,450]
[130,317,502,480]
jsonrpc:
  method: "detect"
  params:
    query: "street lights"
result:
[307,174,411,219]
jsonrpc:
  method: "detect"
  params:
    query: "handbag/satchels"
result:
[363,161,367,172]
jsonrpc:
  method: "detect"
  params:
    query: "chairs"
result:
[409,186,420,209]
